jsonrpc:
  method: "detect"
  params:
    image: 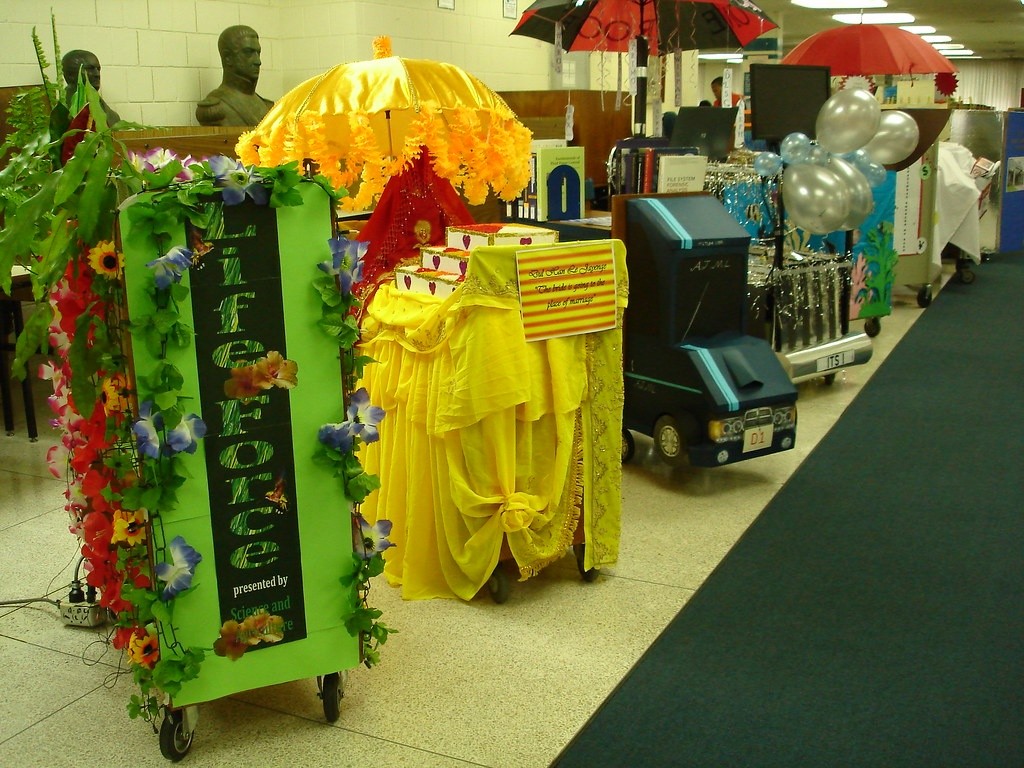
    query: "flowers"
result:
[39,146,399,723]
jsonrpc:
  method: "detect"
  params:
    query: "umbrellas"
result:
[777,9,962,78]
[235,33,534,213]
[507,0,780,139]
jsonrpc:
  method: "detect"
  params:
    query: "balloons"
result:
[860,108,919,166]
[780,129,812,165]
[754,152,782,177]
[814,88,883,154]
[783,146,887,236]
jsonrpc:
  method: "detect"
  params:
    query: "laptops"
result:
[668,106,740,163]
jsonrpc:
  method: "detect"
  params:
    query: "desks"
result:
[0,265,38,442]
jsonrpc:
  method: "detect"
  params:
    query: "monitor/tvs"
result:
[750,63,831,142]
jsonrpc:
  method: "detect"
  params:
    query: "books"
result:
[505,139,586,222]
[614,146,709,196]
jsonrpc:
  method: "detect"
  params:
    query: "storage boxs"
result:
[395,222,558,298]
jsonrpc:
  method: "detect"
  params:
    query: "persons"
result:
[194,24,275,127]
[710,76,747,108]
[62,49,120,129]
